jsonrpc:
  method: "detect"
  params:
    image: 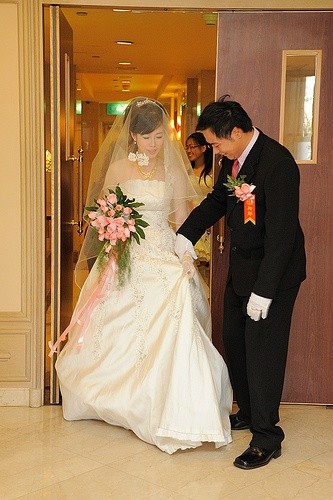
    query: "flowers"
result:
[84,186,150,286]
[223,174,257,203]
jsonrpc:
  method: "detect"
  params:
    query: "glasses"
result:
[186,144,201,150]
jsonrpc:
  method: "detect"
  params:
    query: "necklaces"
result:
[136,153,160,180]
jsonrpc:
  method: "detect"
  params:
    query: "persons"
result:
[47,96,234,453]
[173,95,307,469]
[185,131,213,269]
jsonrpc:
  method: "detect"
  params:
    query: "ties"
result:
[231,160,239,182]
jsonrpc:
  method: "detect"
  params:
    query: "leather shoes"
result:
[234,444,281,470]
[230,413,250,430]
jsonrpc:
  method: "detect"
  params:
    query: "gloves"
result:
[245,292,273,321]
[174,232,198,262]
